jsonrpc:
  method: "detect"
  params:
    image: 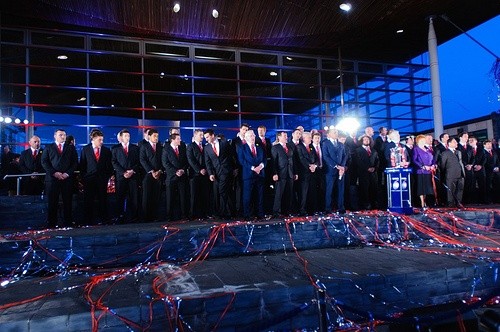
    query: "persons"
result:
[66,135,76,147]
[41,128,78,229]
[18,135,44,196]
[79,124,500,226]
[0,146,21,196]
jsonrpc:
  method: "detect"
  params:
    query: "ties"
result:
[124,145,126,153]
[489,150,492,157]
[316,146,319,156]
[59,144,62,152]
[174,147,179,156]
[33,149,37,158]
[284,146,289,154]
[453,149,458,157]
[153,144,155,150]
[96,146,100,160]
[474,147,476,155]
[251,144,256,155]
[198,142,202,150]
[213,143,216,152]
[367,146,370,155]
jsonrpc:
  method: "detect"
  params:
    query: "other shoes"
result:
[457,203,464,208]
[272,213,282,217]
[257,216,265,222]
[245,216,252,221]
